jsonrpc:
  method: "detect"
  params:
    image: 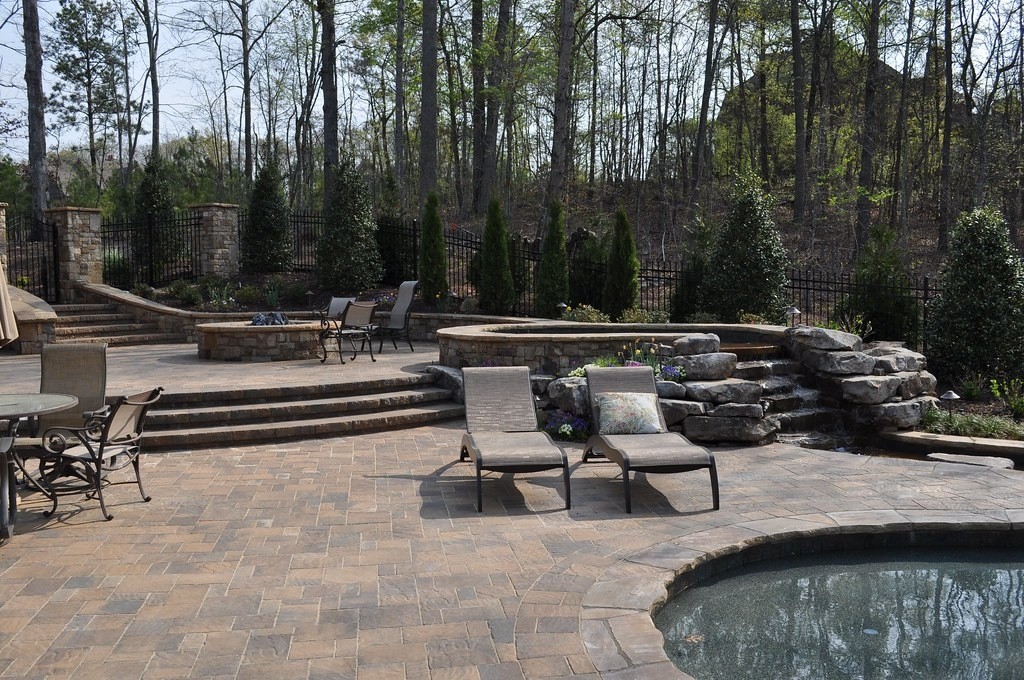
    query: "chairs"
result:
[459,366,570,513]
[312,279,418,365]
[0,342,164,523]
[581,366,720,514]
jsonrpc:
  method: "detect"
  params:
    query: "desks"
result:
[0,392,78,548]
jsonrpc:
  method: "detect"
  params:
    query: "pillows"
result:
[593,392,662,434]
